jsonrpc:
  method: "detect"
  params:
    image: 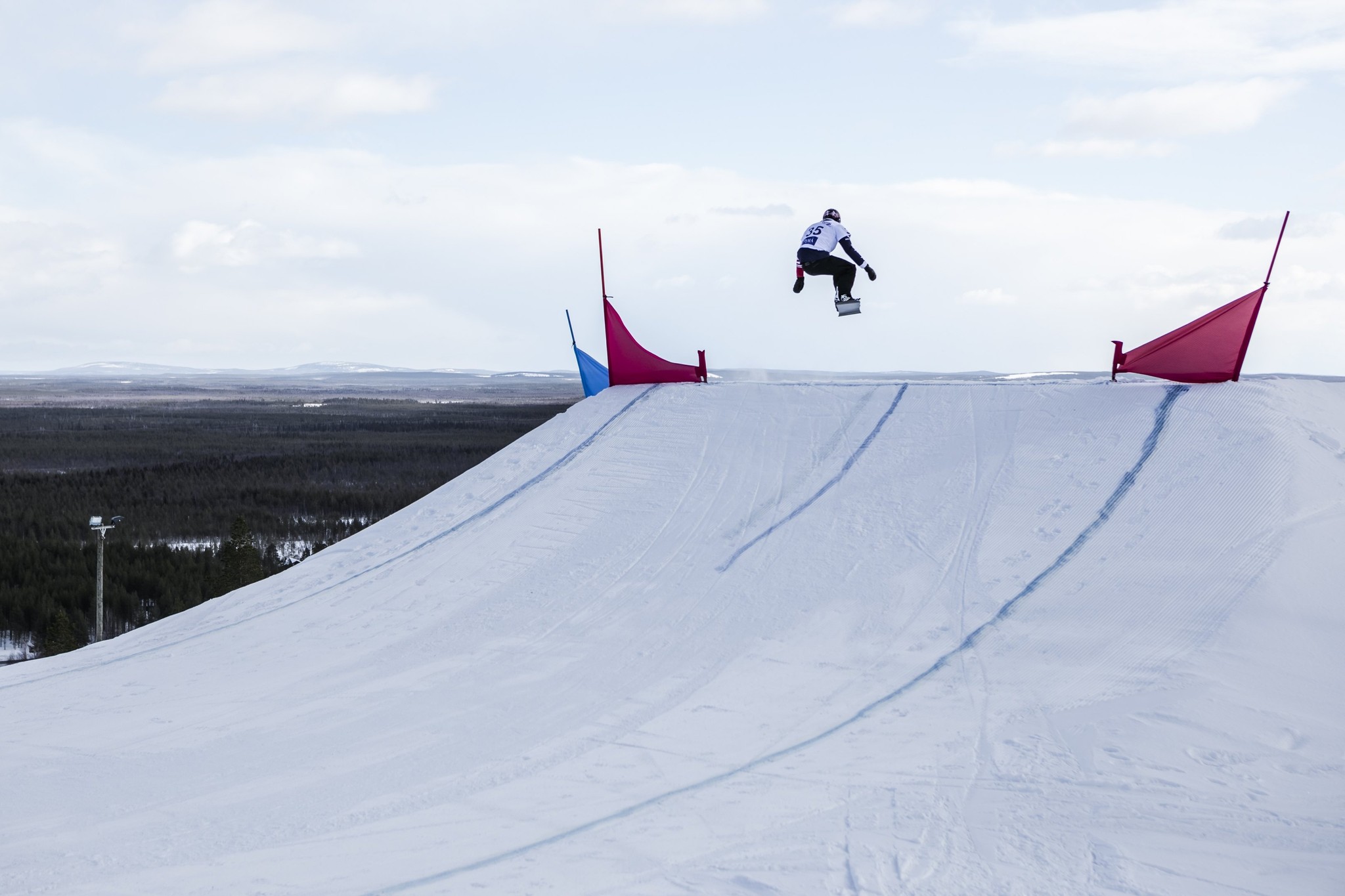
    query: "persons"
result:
[793,209,876,311]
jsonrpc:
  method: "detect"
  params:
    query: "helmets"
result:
[823,208,841,223]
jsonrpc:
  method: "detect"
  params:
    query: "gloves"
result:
[864,264,876,280]
[793,276,804,293]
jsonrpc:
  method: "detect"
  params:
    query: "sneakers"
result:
[834,291,840,303]
[838,294,855,301]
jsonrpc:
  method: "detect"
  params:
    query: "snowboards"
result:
[836,297,863,317]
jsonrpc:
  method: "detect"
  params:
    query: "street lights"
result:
[89,516,124,643]
